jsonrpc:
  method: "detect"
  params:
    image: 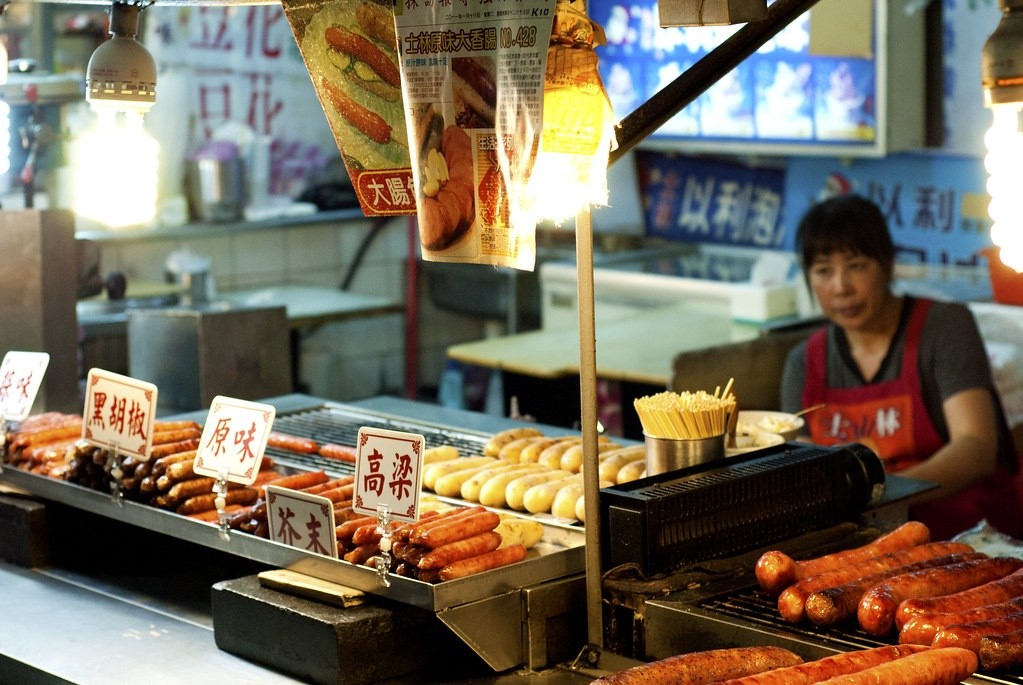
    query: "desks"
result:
[552,302,755,442]
[73,280,403,396]
[445,312,640,430]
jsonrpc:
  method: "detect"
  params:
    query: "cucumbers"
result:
[326,46,353,72]
[354,60,382,81]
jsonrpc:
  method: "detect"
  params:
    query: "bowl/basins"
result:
[737,410,805,442]
[725,431,784,458]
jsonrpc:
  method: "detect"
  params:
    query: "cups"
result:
[189,159,244,204]
[165,272,216,304]
[642,431,725,479]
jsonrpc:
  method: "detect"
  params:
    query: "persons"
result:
[779,193,1021,542]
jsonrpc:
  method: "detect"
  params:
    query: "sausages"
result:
[416,56,497,246]
[6,412,648,583]
[323,8,400,145]
[588,521,1023,685]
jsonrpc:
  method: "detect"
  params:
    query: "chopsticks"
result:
[633,378,737,438]
